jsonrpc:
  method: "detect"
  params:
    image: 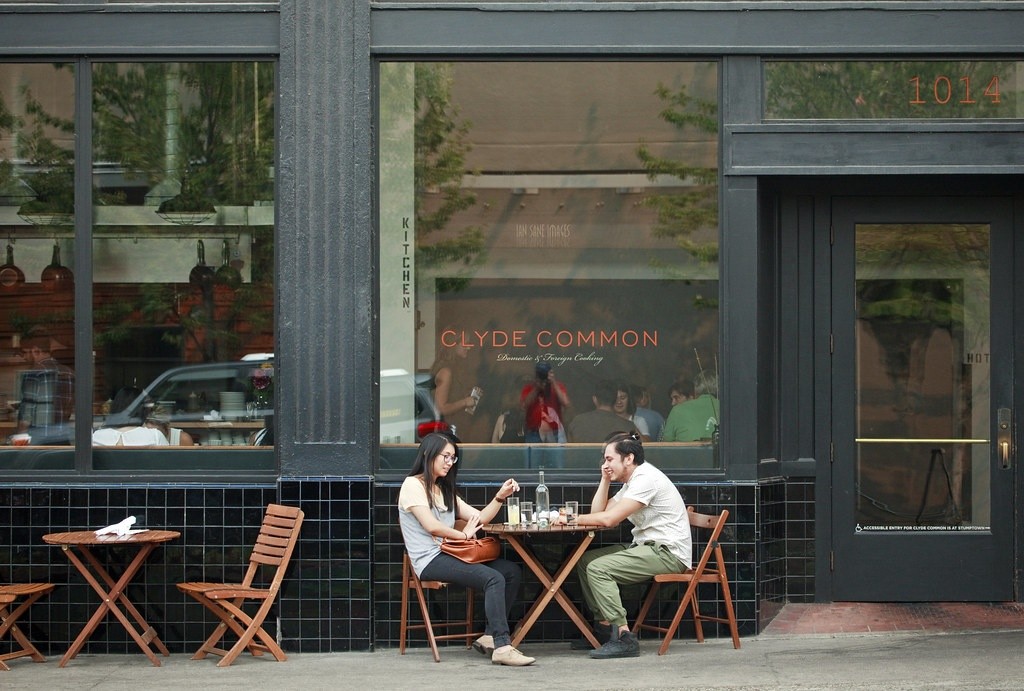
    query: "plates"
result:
[219,392,245,422]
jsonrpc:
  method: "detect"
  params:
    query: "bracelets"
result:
[494,493,506,504]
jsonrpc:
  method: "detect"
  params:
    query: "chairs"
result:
[176,501,306,667]
[0,580,55,671]
[400,546,476,660]
[632,508,740,652]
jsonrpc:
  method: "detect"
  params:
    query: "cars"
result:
[6,354,463,450]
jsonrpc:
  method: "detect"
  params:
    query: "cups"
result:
[520,502,532,524]
[507,497,519,525]
[565,501,578,527]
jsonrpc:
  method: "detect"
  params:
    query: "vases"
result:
[253,391,275,421]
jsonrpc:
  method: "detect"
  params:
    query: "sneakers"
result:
[571,625,641,658]
[473,635,536,666]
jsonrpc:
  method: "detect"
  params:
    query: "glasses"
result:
[438,452,459,465]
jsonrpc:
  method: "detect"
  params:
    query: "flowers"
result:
[246,364,274,399]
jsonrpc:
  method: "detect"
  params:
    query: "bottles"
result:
[535,472,549,527]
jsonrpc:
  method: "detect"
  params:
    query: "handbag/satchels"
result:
[440,536,501,564]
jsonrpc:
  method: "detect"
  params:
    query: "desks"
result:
[41,527,180,668]
[484,520,620,651]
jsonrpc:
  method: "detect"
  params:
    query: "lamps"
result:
[154,162,218,225]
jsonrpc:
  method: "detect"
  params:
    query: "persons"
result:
[548,433,693,658]
[92,385,195,446]
[17,318,75,446]
[431,323,720,443]
[397,433,536,667]
